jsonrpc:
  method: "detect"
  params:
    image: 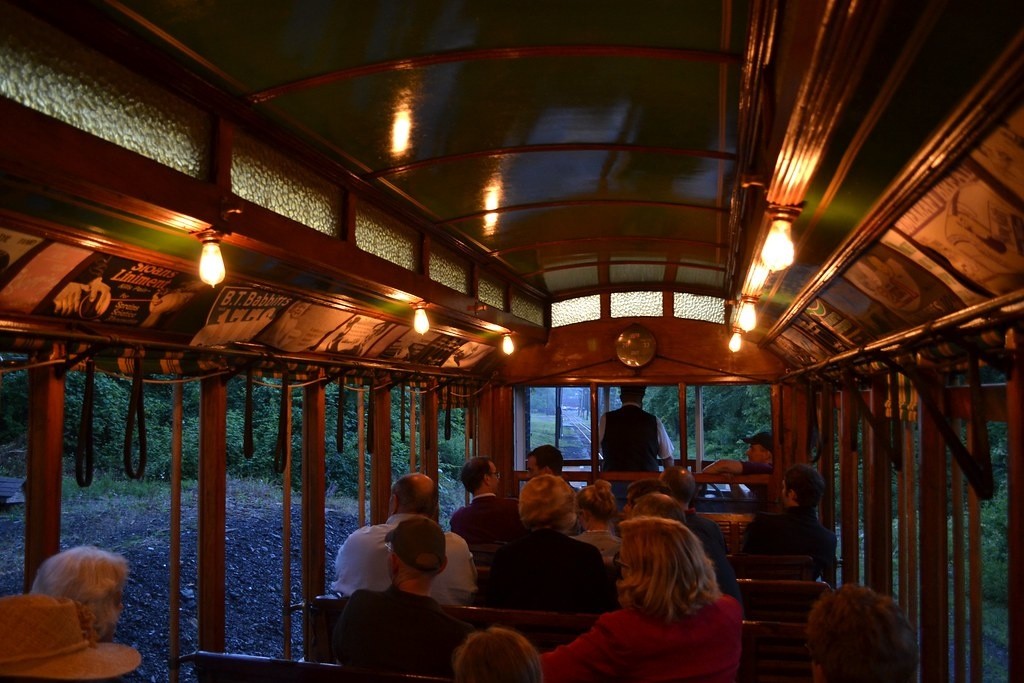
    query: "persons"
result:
[597,386,674,512]
[657,467,746,620]
[803,580,919,683]
[567,479,639,557]
[702,432,773,498]
[537,517,743,683]
[622,478,673,517]
[336,473,478,605]
[0,545,142,683]
[449,456,533,567]
[331,513,477,677]
[489,474,614,614]
[527,444,580,492]
[452,622,543,683]
[742,465,838,581]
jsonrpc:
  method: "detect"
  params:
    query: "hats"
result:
[519,473,576,528]
[741,431,773,451]
[0,594,142,680]
[385,515,446,571]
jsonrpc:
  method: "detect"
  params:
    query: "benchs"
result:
[177,513,830,683]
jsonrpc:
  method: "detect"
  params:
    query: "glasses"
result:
[485,472,500,479]
[384,542,394,552]
[612,551,631,569]
